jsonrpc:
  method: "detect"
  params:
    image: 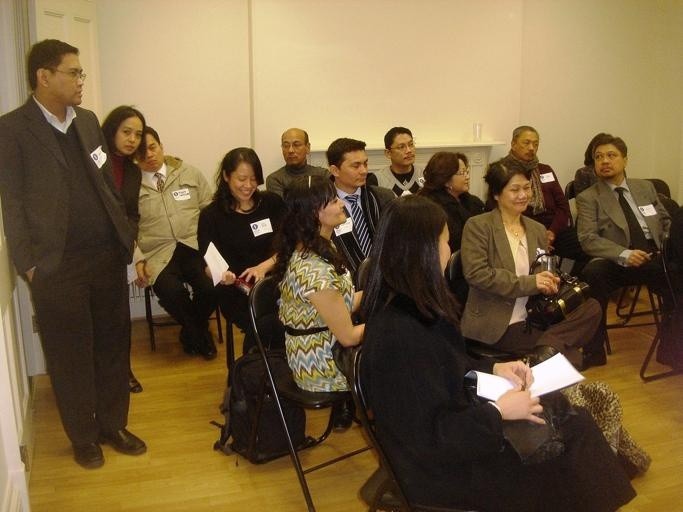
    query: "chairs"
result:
[145,179,683,512]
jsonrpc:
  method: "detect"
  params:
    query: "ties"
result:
[155,172,165,192]
[345,194,373,259]
[614,186,649,251]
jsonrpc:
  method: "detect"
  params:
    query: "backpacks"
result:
[209,345,317,465]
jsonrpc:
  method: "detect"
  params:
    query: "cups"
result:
[539,254,558,278]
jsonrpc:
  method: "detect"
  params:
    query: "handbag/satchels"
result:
[524,253,594,335]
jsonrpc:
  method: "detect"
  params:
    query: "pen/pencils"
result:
[520,356,531,391]
[645,252,653,257]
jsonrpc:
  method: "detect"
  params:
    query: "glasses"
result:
[388,141,417,152]
[44,66,87,83]
[452,168,470,177]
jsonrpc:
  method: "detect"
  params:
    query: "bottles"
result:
[234,277,252,296]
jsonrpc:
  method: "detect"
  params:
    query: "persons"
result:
[197,125,487,432]
[459,161,601,369]
[128,127,220,359]
[101,106,147,392]
[574,134,683,371]
[500,124,590,273]
[0,39,148,469]
[359,197,638,511]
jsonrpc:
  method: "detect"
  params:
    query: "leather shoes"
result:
[129,370,143,393]
[333,400,355,431]
[580,345,607,373]
[199,339,217,360]
[178,328,199,354]
[97,427,147,456]
[657,346,683,373]
[72,439,105,469]
